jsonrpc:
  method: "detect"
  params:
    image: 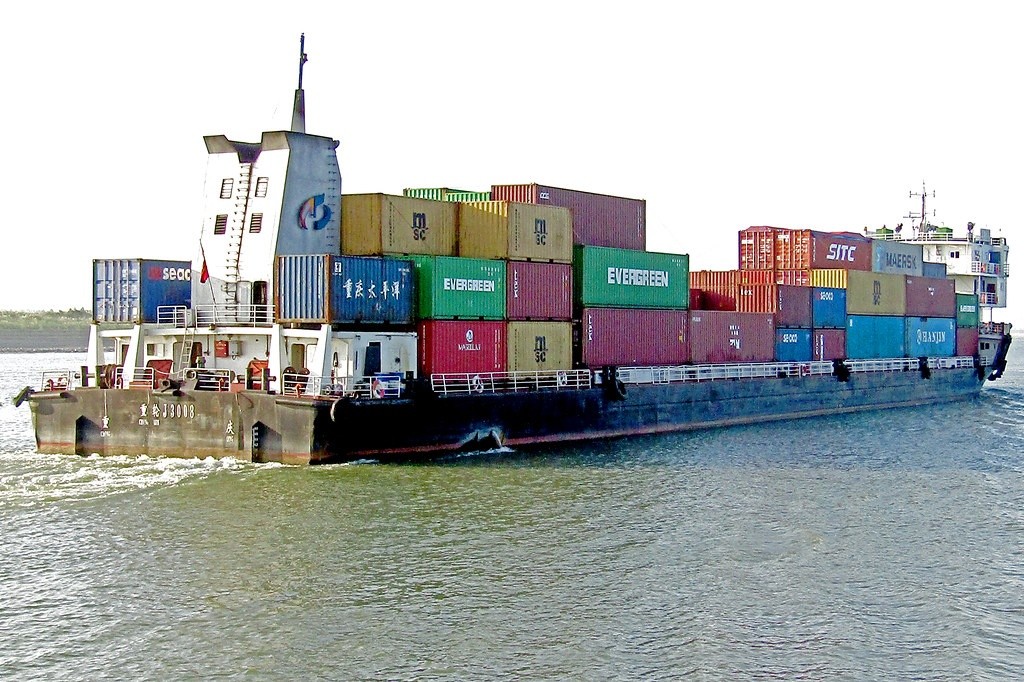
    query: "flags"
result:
[200,261,209,283]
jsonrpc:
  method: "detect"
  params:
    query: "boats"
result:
[10,30,1014,468]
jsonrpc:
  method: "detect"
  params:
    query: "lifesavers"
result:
[977,366,985,379]
[57,374,70,386]
[981,294,986,303]
[995,265,998,274]
[472,376,484,394]
[936,358,941,369]
[372,379,385,399]
[610,378,628,400]
[558,371,568,386]
[801,364,807,377]
[834,362,851,382]
[981,263,985,272]
[334,385,343,395]
[993,296,999,303]
[921,363,931,379]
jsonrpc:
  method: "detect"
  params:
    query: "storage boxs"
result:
[88,181,1000,393]
